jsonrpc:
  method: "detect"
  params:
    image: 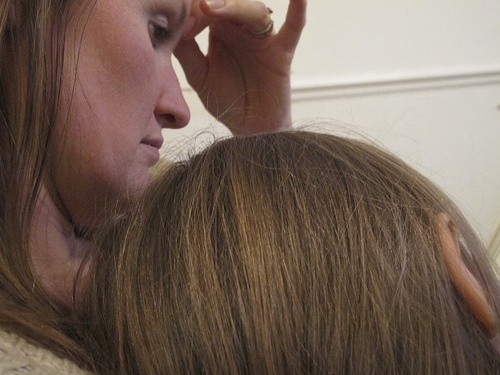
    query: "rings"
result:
[248,6,273,39]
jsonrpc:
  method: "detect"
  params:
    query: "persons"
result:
[0,0,307,375]
[78,126,498,375]
